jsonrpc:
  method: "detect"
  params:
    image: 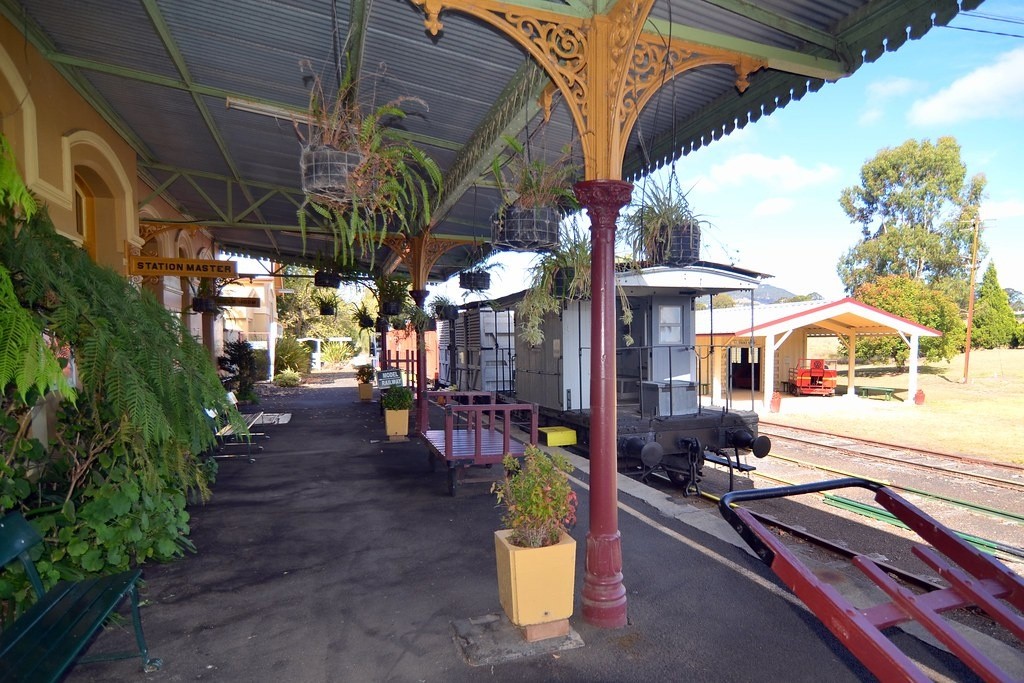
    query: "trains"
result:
[386,259,775,497]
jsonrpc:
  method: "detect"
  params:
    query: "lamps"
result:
[192,276,217,313]
[281,231,342,243]
[223,97,362,135]
[274,287,294,293]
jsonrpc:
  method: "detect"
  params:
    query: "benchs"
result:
[861,386,896,403]
[205,391,264,462]
[0,513,148,682]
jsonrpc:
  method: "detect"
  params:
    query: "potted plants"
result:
[305,85,703,333]
[217,339,262,405]
[381,383,415,441]
[357,365,375,398]
[486,445,581,642]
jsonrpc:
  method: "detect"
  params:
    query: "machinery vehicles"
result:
[788,359,837,397]
